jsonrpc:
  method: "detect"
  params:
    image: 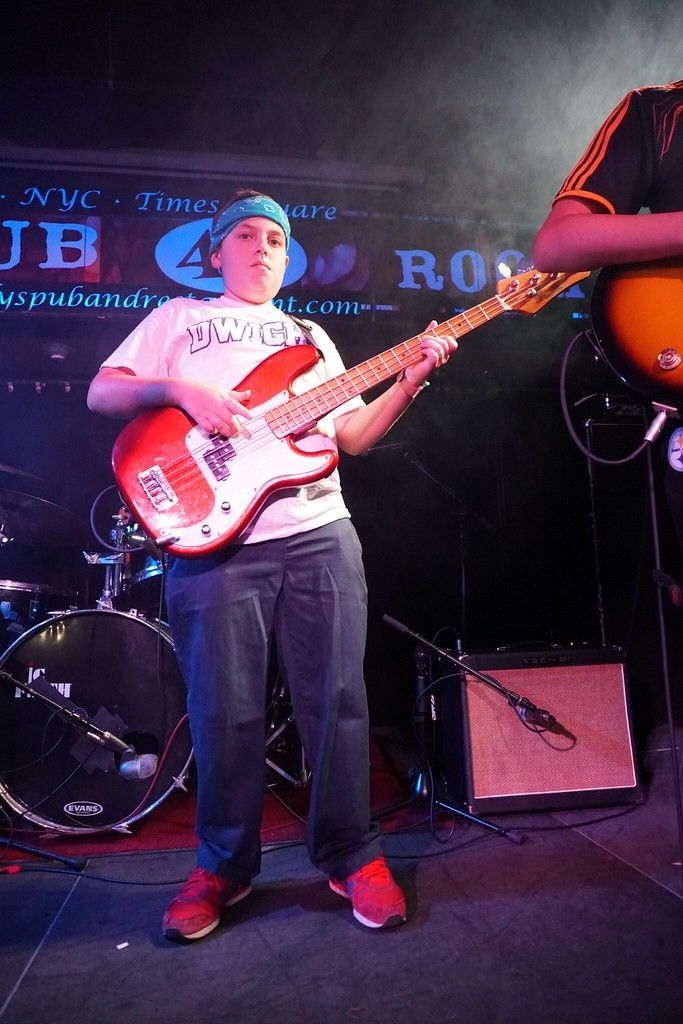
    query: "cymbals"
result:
[0,490,80,523]
[1,465,47,486]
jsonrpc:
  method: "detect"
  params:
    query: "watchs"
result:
[397,369,431,398]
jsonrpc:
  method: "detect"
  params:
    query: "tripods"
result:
[263,711,312,791]
[372,613,550,846]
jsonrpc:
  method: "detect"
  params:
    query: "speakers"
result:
[458,645,644,816]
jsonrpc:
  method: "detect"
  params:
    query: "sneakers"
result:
[329,851,406,928]
[160,862,252,941]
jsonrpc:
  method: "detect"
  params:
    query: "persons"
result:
[88,189,458,941]
[532,80,682,272]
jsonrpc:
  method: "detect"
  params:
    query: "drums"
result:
[112,563,168,622]
[0,607,199,840]
[0,580,55,637]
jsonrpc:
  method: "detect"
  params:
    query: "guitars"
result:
[108,263,594,564]
[580,261,682,426]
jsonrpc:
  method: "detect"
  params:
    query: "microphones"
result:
[518,707,556,722]
[119,748,158,780]
[360,441,413,459]
